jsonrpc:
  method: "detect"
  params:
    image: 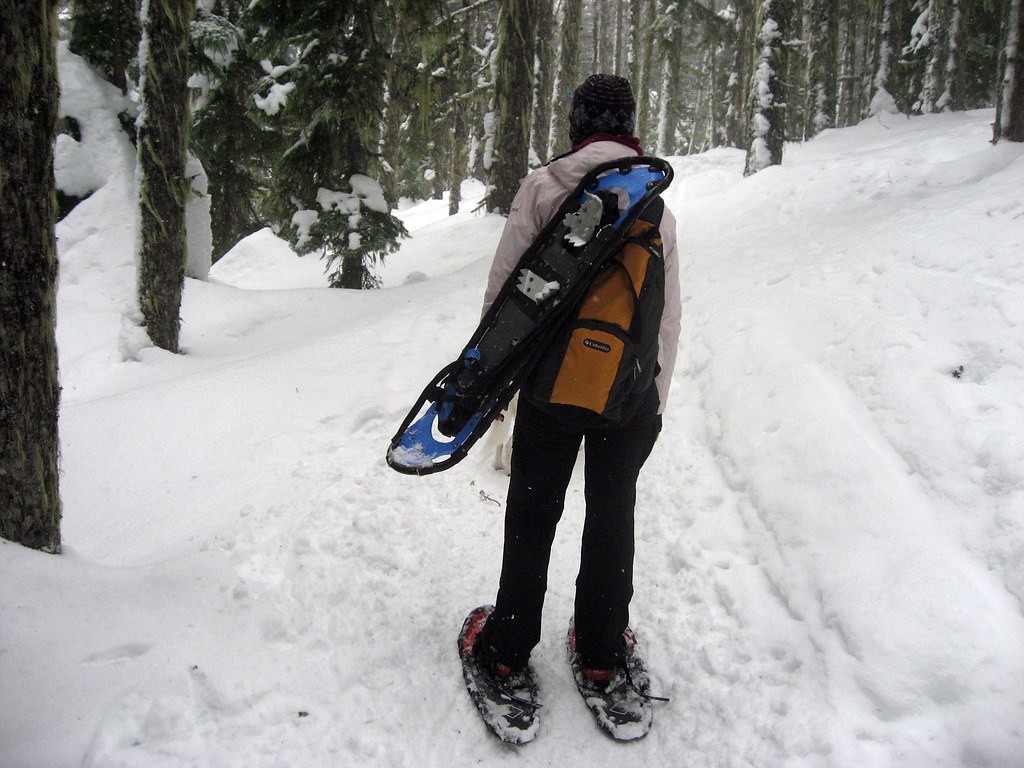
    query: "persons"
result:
[471,75,682,687]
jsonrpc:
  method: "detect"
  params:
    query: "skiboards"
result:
[454,603,652,744]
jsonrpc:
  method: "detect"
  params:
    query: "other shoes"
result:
[460,609,510,677]
[568,626,635,683]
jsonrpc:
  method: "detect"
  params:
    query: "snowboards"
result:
[386,154,674,478]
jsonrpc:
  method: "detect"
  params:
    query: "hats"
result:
[568,73,636,144]
